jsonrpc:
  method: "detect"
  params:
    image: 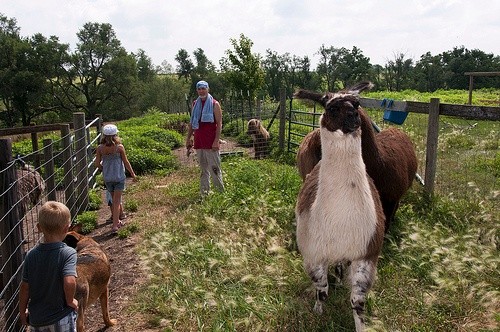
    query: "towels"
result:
[190,93,214,129]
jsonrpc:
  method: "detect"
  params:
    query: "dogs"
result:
[247,119,270,159]
[62,223,117,332]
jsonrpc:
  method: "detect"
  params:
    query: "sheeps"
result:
[295,81,419,332]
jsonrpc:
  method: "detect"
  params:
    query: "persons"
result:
[18,201,78,332]
[106,190,127,221]
[95,123,138,233]
[185,81,225,197]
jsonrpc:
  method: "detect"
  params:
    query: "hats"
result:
[196,81,208,89]
[102,124,120,135]
